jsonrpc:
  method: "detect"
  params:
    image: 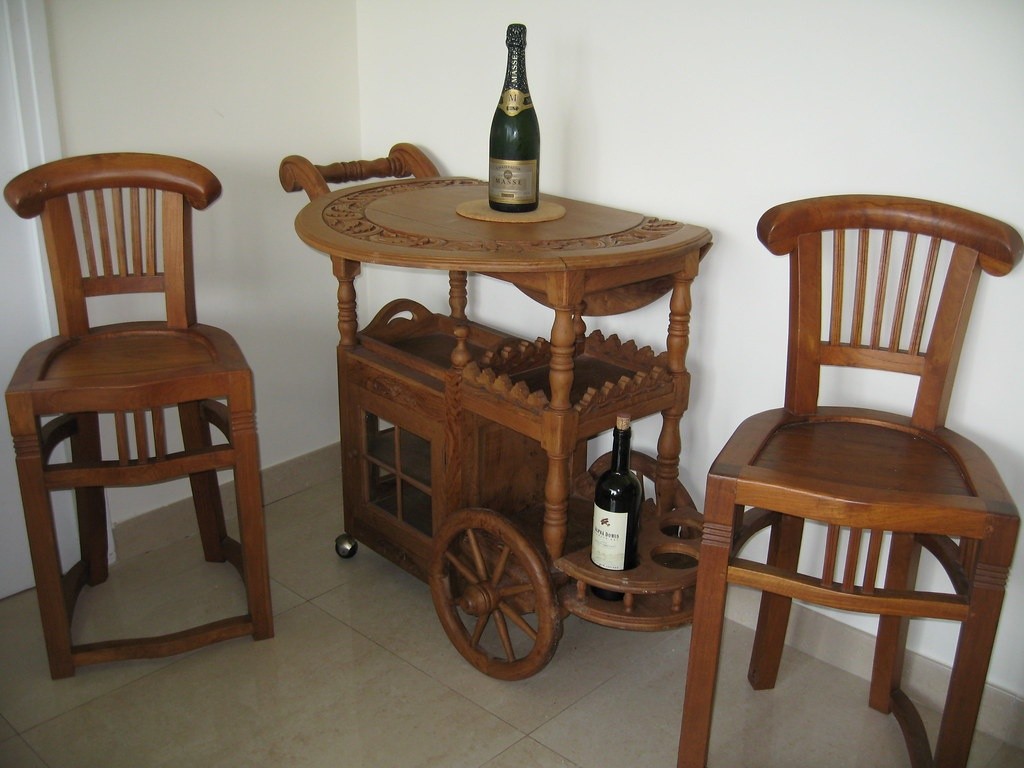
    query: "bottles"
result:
[590,413,642,600]
[489,24,541,213]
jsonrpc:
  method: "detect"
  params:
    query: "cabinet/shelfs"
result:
[278,143,713,682]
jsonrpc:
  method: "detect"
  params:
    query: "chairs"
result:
[2,152,275,682]
[675,194,1024,768]
